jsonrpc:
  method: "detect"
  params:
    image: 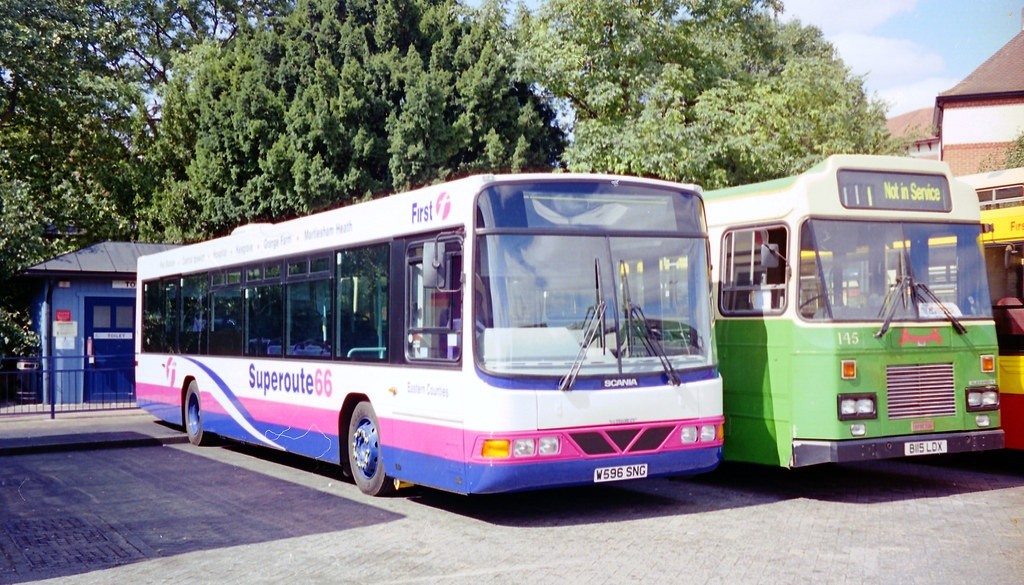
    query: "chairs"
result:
[249,336,334,359]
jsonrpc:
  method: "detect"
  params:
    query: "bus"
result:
[702,152,1007,479]
[511,165,1024,463]
[134,172,725,495]
